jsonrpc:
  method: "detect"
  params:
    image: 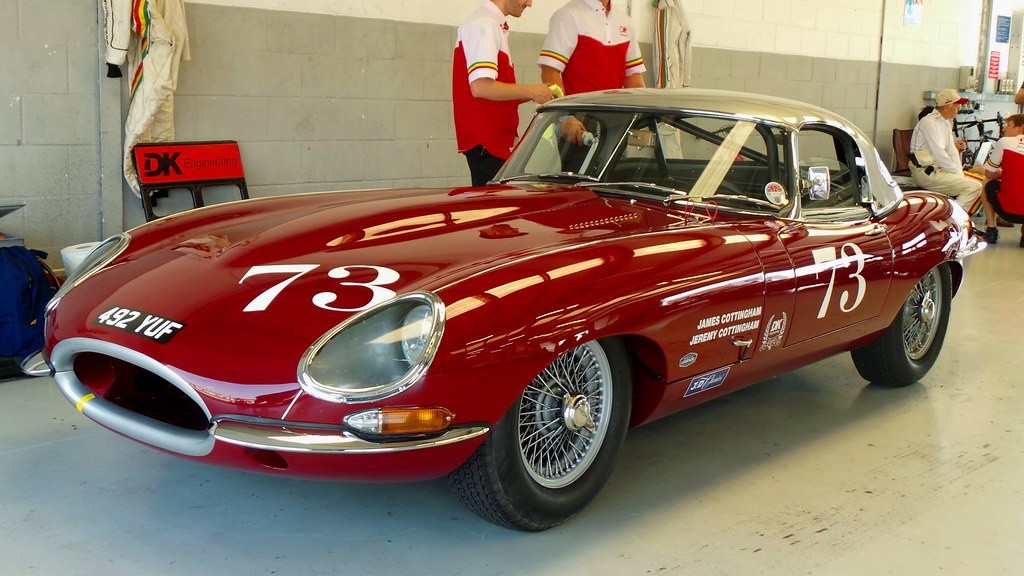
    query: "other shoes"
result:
[981,230,998,244]
[985,215,1014,227]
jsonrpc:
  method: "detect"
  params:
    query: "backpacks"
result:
[0,245,60,357]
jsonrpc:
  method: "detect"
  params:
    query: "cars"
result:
[47,87,988,532]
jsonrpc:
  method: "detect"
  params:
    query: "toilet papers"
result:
[59,242,101,278]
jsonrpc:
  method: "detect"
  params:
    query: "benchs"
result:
[606,156,790,207]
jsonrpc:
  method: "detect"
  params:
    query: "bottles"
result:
[555,124,595,147]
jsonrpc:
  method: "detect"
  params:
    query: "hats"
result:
[936,88,968,106]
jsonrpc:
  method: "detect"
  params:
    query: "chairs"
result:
[892,129,925,191]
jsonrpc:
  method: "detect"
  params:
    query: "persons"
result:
[981,114,1024,247]
[1014,82,1024,104]
[453,0,558,187]
[908,89,982,213]
[537,0,647,173]
